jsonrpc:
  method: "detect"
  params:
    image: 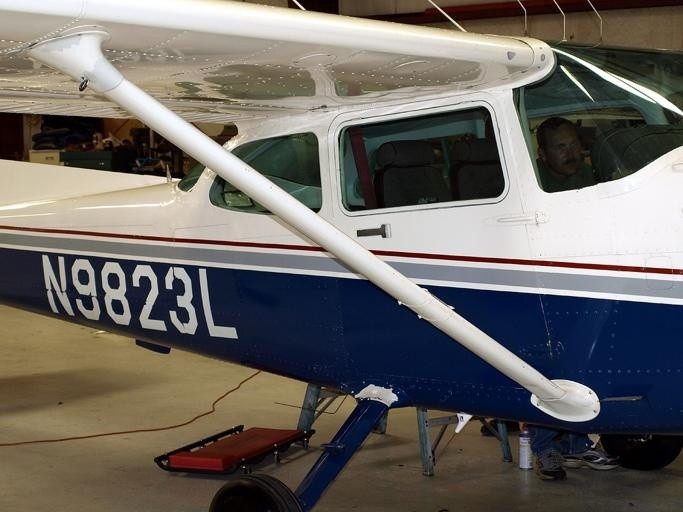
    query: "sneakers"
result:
[535,448,568,481]
[482,419,519,435]
[564,443,620,470]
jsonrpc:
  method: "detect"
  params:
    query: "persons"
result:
[527,425,619,481]
[535,116,601,193]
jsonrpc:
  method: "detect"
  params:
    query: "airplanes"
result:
[0,0,683,512]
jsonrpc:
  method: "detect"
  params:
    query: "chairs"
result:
[373,138,505,209]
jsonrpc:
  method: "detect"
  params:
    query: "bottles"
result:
[519,430,534,470]
